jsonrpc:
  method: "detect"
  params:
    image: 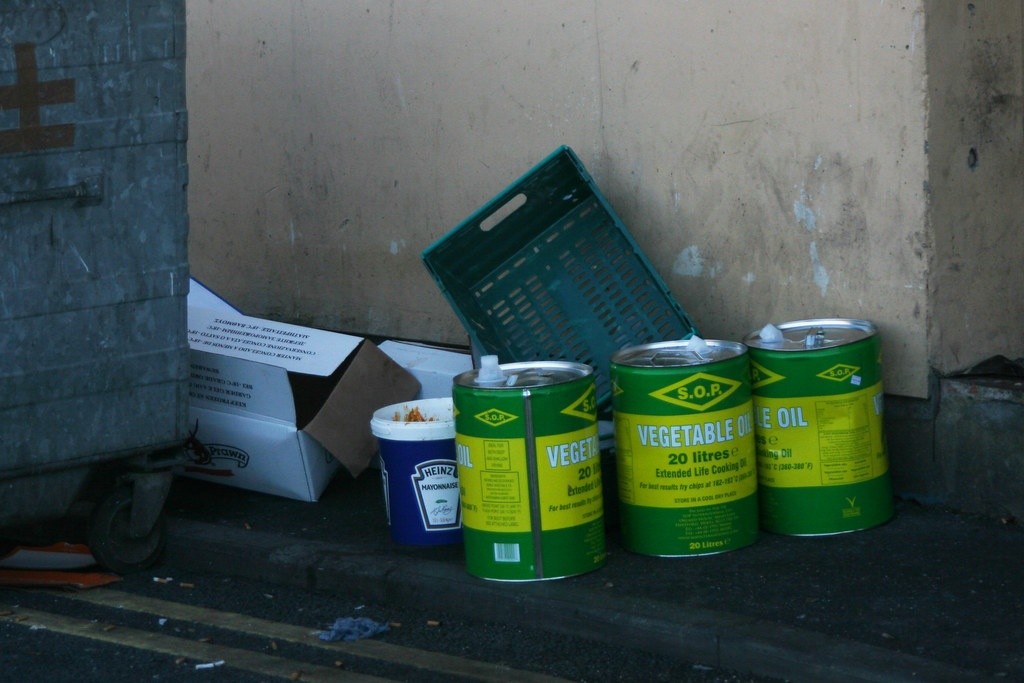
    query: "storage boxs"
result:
[179,304,422,501]
[366,333,475,401]
[418,145,696,412]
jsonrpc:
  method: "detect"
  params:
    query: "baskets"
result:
[423,146,702,418]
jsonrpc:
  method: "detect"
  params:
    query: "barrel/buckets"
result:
[370,396,463,546]
